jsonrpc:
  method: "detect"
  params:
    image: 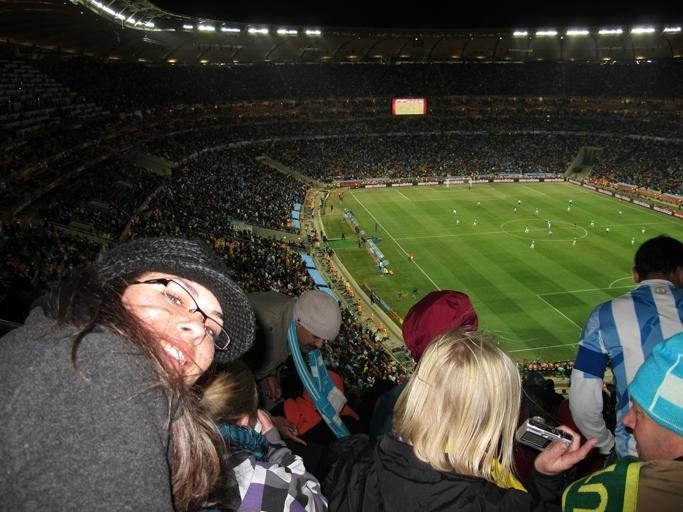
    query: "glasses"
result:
[123,278,230,352]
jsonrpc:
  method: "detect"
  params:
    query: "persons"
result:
[443,177,646,249]
[559,332,683,512]
[2,171,414,399]
[1,52,590,176]
[369,290,530,491]
[268,372,361,482]
[189,362,329,512]
[242,291,342,446]
[586,59,682,207]
[318,328,598,511]
[0,237,255,512]
[567,234,682,467]
[516,358,577,380]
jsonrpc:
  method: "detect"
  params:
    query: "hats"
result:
[403,291,478,362]
[91,239,255,363]
[628,331,683,435]
[296,290,341,341]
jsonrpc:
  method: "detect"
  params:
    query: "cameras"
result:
[515,416,574,453]
